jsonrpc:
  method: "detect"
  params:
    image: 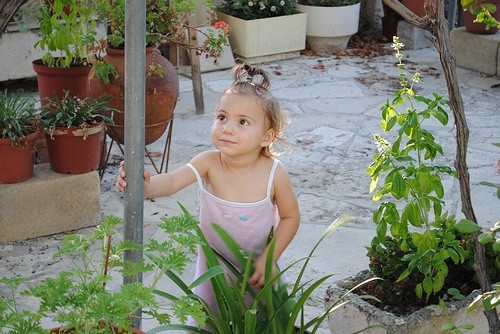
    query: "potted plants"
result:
[295,0,361,52]
[0,214,206,334]
[0,87,124,184]
[325,36,500,334]
[461,0,500,34]
[14,0,98,111]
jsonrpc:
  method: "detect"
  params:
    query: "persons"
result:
[116,58,301,334]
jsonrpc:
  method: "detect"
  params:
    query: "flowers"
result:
[86,0,231,85]
[217,0,300,20]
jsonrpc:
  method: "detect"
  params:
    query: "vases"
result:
[401,0,436,18]
[217,10,308,65]
[87,45,178,146]
